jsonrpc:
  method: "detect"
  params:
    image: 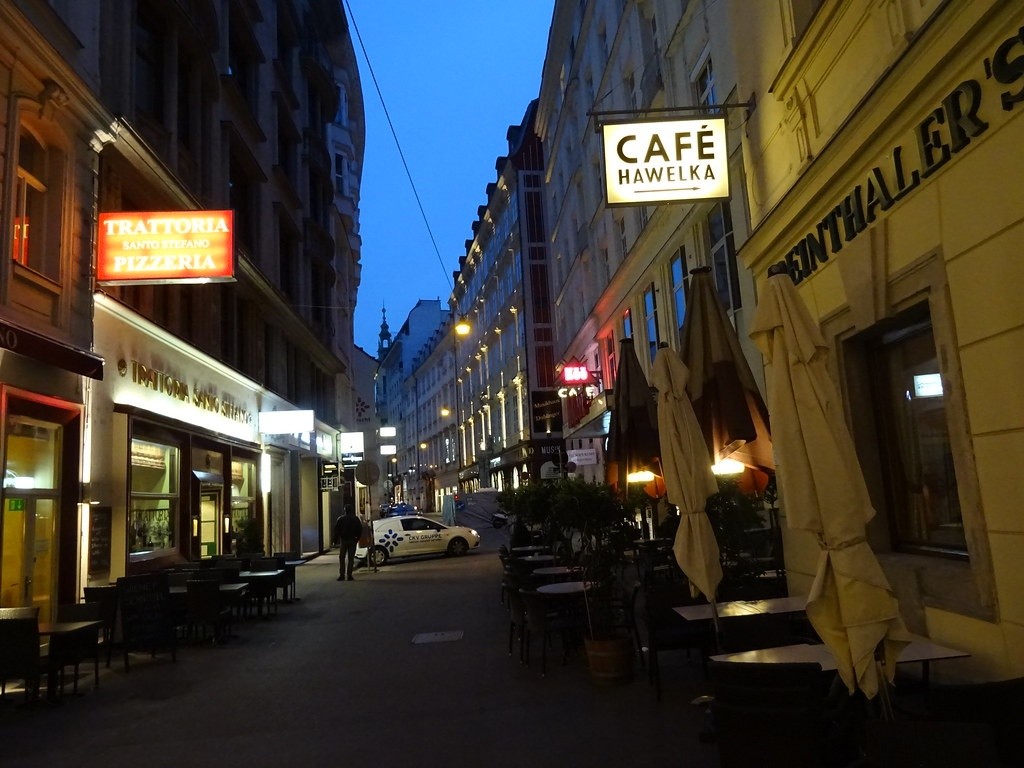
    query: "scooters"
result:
[492,508,511,528]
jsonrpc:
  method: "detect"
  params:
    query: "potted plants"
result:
[557,491,643,681]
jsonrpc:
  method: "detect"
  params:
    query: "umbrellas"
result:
[675,266,773,457]
[654,344,727,656]
[749,273,914,719]
[609,339,661,474]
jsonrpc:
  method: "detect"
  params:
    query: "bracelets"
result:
[642,647,648,651]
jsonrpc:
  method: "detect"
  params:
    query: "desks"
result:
[36,621,102,698]
[280,559,307,602]
[238,569,284,616]
[511,536,972,723]
[170,582,248,645]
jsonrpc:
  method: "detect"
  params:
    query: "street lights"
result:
[392,457,398,477]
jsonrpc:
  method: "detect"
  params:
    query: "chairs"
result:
[497,545,646,679]
[948,676,1023,768]
[0,549,300,706]
[709,662,840,768]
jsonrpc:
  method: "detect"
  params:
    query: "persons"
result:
[544,526,568,543]
[510,522,534,555]
[333,504,362,580]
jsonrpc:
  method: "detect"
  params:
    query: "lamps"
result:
[454,313,470,335]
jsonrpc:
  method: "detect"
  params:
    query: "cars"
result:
[379,503,390,517]
[354,515,480,567]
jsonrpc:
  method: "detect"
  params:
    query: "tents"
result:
[443,488,512,548]
